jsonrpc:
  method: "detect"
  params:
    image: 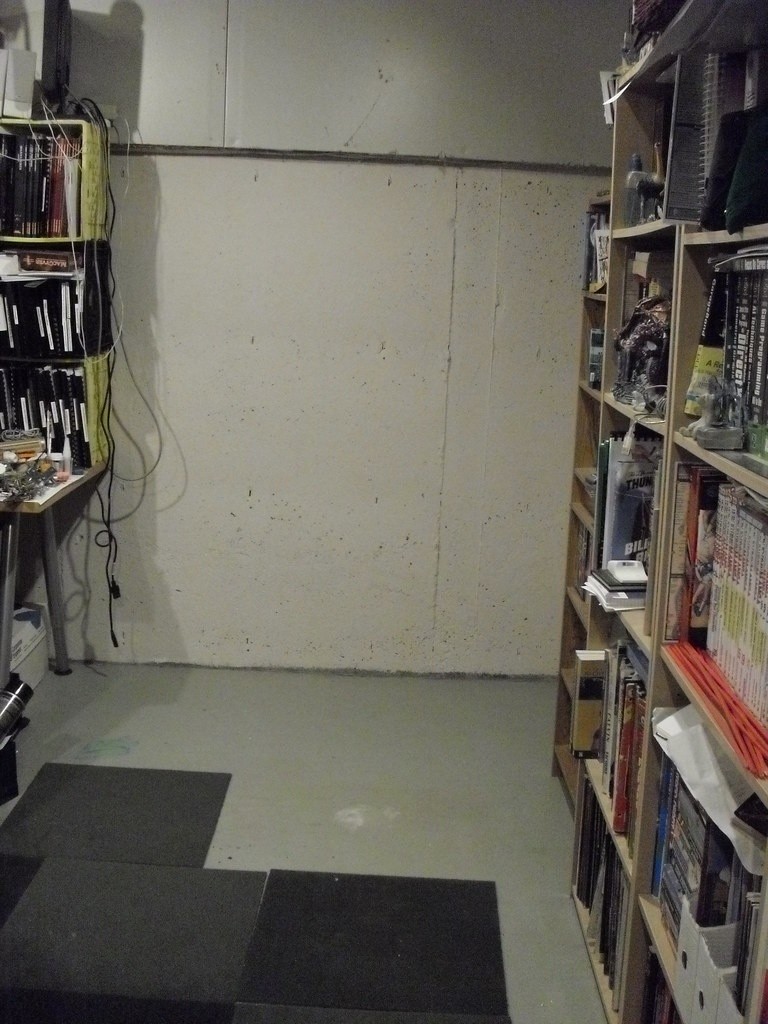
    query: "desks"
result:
[0,459,111,677]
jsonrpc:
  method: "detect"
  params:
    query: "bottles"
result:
[0,672,34,743]
[622,154,655,228]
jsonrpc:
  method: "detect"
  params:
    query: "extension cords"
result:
[45,112,94,122]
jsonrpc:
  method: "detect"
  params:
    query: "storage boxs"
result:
[0,351,111,466]
[0,244,112,359]
[10,601,50,689]
[0,117,111,243]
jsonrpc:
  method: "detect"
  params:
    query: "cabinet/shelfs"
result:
[549,0,768,1024]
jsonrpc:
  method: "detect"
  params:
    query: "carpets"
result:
[0,763,512,1024]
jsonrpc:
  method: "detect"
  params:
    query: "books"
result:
[572,634,768,1024]
[577,86,768,730]
[0,280,91,469]
[0,133,81,238]
